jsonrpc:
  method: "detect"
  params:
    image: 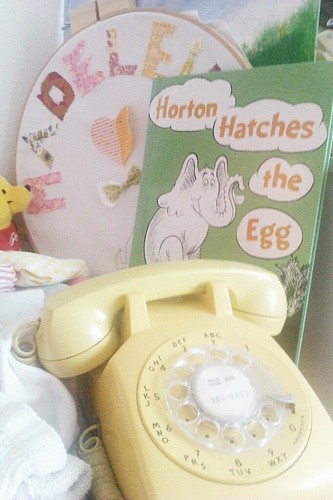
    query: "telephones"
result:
[34,259,333,500]
